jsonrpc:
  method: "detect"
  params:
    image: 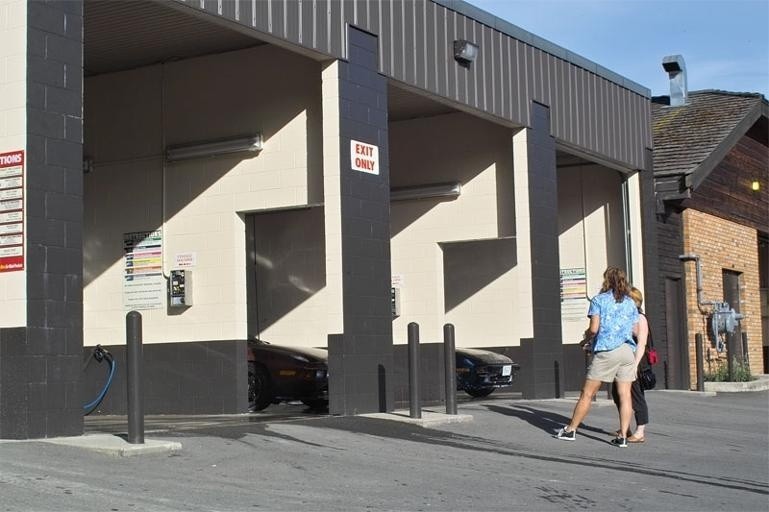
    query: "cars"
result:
[247,334,327,413]
[453,346,519,397]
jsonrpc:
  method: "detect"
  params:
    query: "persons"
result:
[556,266,640,447]
[612,288,651,443]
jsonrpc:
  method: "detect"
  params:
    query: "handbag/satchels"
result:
[645,347,659,365]
[641,367,656,389]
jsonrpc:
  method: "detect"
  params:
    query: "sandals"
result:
[611,428,631,437]
[627,433,645,442]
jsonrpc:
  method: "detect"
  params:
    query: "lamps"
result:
[454,39,479,64]
[750,174,759,192]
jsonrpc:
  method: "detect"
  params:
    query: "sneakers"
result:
[551,426,576,442]
[610,436,628,448]
